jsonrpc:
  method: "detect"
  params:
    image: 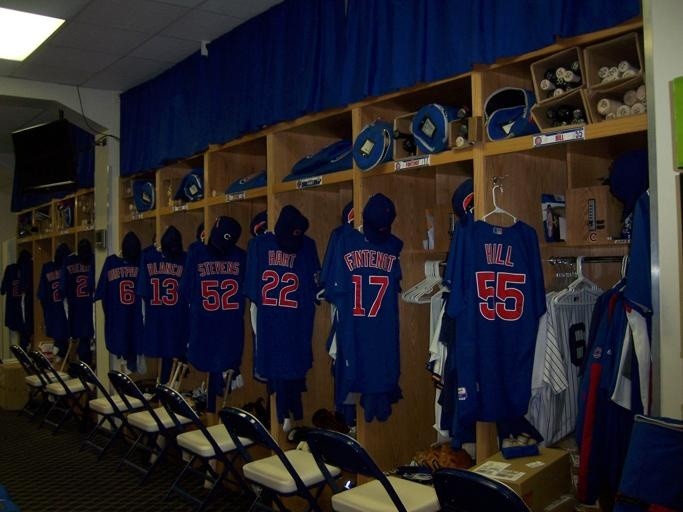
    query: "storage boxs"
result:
[1,358,28,411]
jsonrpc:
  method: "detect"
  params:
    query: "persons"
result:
[543,204,559,243]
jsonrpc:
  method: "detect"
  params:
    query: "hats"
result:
[18,250,33,267]
[275,205,309,254]
[122,231,141,262]
[55,244,72,267]
[211,215,241,253]
[161,226,182,257]
[79,238,92,260]
[362,193,396,245]
[610,150,647,222]
